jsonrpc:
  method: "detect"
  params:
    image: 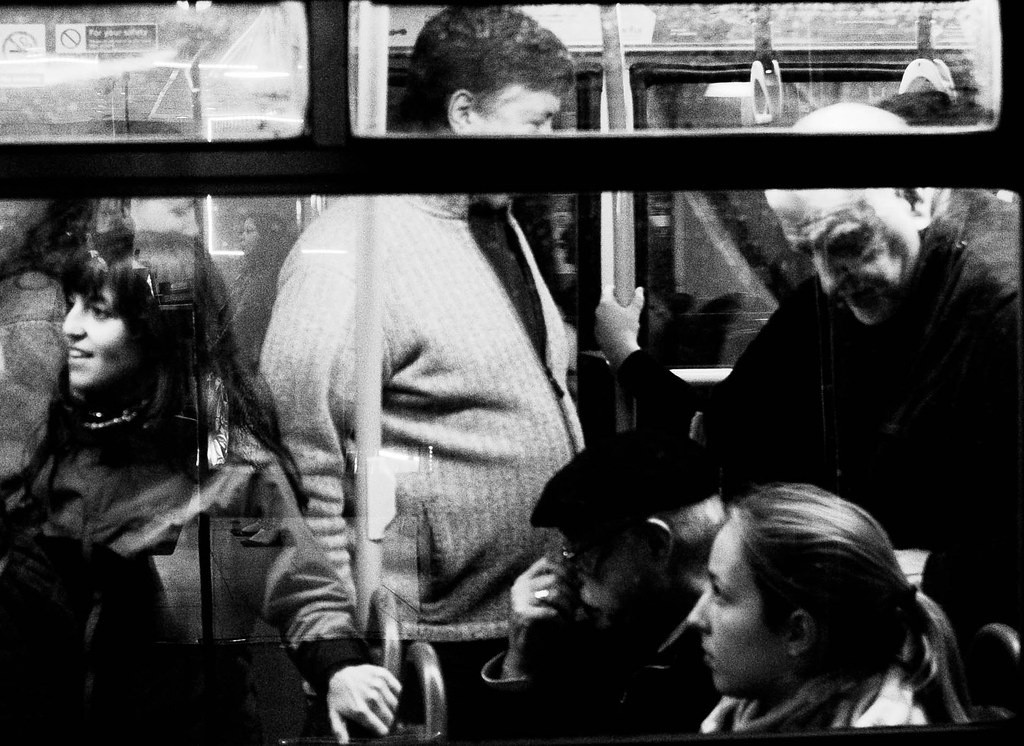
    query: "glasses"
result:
[557,528,644,576]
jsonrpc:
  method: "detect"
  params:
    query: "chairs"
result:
[361,588,454,744]
[961,621,1022,730]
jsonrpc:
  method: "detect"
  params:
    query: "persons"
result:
[0,0,1024,746]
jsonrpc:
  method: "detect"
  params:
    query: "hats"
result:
[531,423,719,554]
